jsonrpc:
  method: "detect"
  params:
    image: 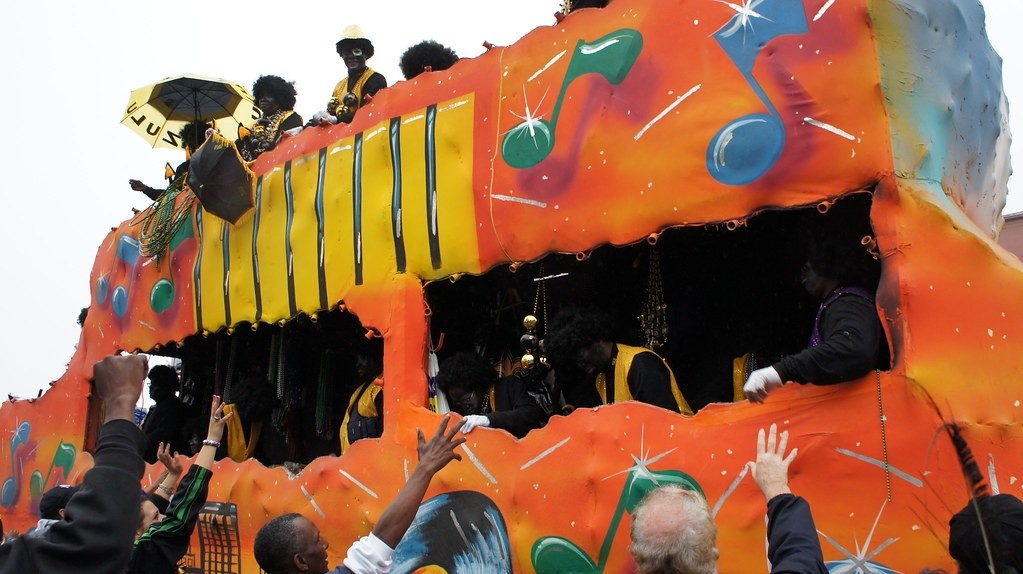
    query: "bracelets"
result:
[202,440,220,447]
[159,484,173,495]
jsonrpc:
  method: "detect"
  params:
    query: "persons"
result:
[434,350,545,439]
[543,316,694,417]
[128,120,214,200]
[328,39,387,123]
[222,339,385,474]
[716,226,890,406]
[400,42,459,81]
[235,75,303,161]
[0,354,1023,574]
[140,364,201,464]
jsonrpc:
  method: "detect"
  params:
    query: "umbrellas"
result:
[121,72,264,149]
[184,132,258,226]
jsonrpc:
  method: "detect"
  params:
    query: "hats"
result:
[336,26,375,59]
[40,484,81,519]
[949,494,1023,574]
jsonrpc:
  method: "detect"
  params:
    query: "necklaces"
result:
[481,389,491,414]
[263,108,285,134]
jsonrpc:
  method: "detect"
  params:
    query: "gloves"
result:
[459,415,490,434]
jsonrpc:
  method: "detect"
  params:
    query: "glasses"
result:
[451,391,475,409]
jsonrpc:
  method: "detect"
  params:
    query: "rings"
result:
[215,417,219,420]
